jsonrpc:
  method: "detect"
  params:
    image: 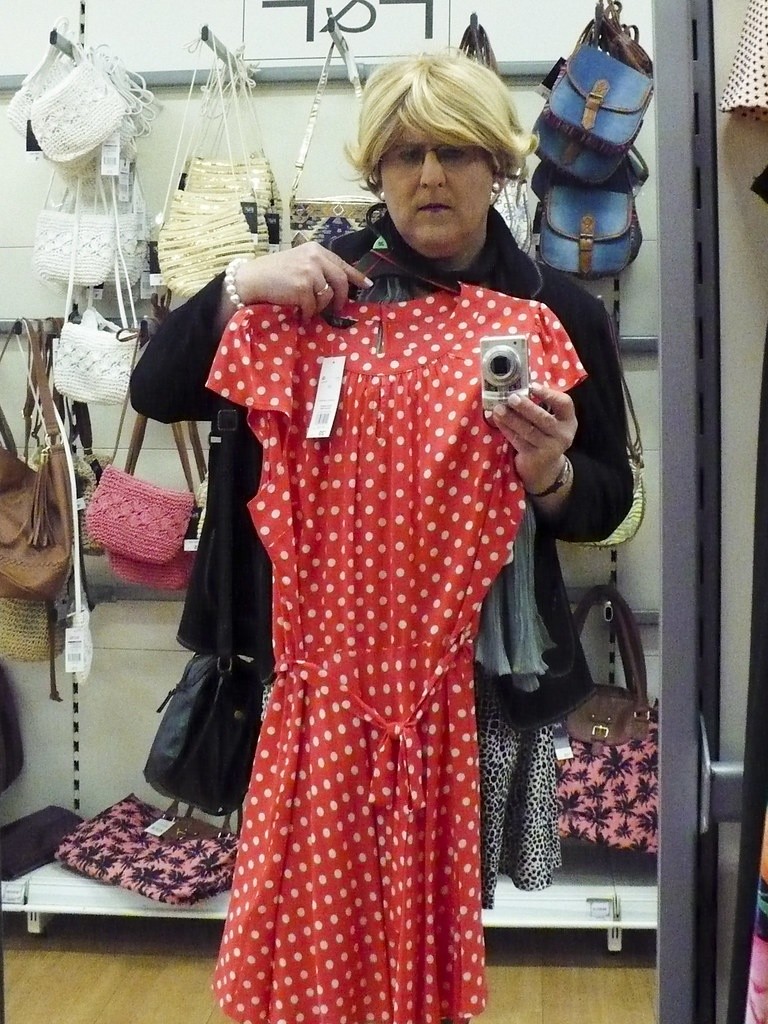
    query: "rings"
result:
[314,283,329,296]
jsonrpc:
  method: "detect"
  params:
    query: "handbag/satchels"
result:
[547,581,658,857]
[452,0,653,546]
[143,649,264,818]
[2,31,387,662]
[53,793,245,904]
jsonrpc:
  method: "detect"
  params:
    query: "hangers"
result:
[348,203,461,294]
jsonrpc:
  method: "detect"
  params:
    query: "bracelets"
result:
[225,259,247,309]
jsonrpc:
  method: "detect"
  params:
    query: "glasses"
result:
[387,143,483,171]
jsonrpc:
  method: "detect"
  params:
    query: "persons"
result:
[129,51,637,1024]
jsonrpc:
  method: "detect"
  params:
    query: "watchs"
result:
[525,456,571,497]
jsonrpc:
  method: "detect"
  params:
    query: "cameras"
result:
[481,334,530,410]
